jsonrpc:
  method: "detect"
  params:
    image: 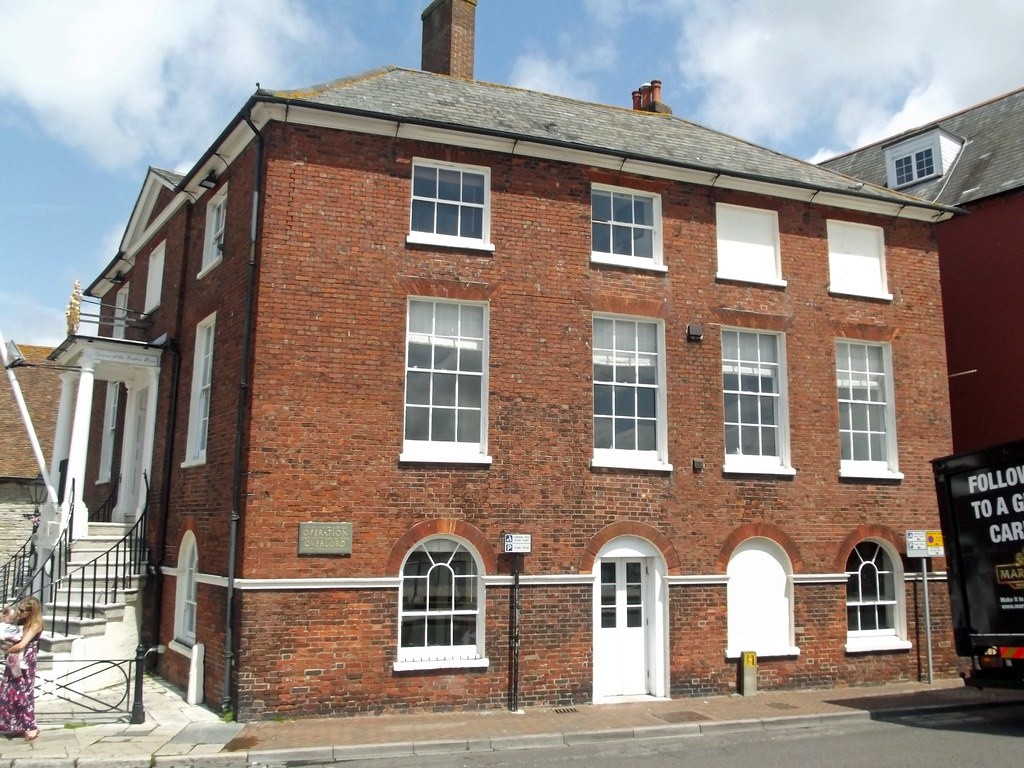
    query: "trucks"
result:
[927,436,1024,690]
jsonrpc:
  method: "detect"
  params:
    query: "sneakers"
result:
[26,728,41,741]
[5,729,24,738]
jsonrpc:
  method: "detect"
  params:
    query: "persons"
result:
[0,596,44,741]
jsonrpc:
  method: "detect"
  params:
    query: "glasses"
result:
[18,606,31,613]
